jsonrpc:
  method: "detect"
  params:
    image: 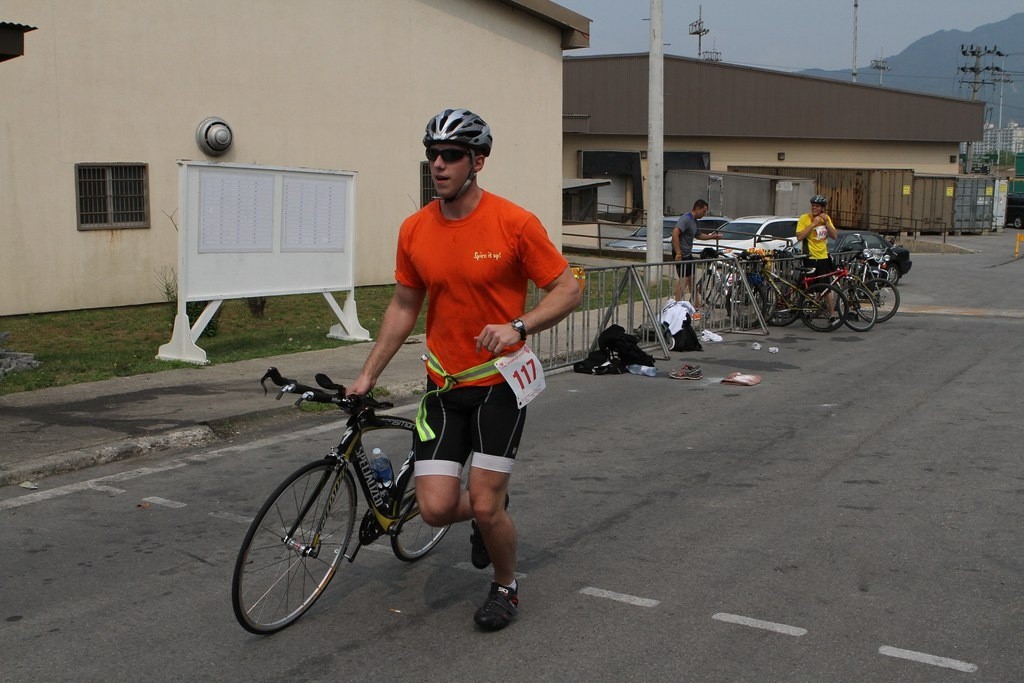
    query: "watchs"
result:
[510,318,528,342]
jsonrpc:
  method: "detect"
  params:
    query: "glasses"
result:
[426,147,469,162]
[811,204,822,208]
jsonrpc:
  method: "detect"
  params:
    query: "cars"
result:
[764,229,913,288]
[1005,195,1024,229]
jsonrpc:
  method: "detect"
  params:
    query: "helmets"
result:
[423,109,493,157]
[810,195,827,207]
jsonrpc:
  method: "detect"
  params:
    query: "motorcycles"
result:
[851,234,905,299]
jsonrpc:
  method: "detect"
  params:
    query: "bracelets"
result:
[676,254,681,257]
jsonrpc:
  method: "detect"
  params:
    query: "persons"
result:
[670,199,723,311]
[346,107,582,629]
[796,194,841,326]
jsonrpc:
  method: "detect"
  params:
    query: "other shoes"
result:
[669,370,700,380]
[672,363,703,378]
[830,317,840,325]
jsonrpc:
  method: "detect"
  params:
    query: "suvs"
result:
[606,215,734,255]
[691,215,803,261]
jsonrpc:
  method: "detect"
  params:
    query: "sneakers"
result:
[474,579,519,631]
[469,493,509,569]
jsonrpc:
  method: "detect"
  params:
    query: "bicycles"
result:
[691,248,901,332]
[230,368,454,636]
